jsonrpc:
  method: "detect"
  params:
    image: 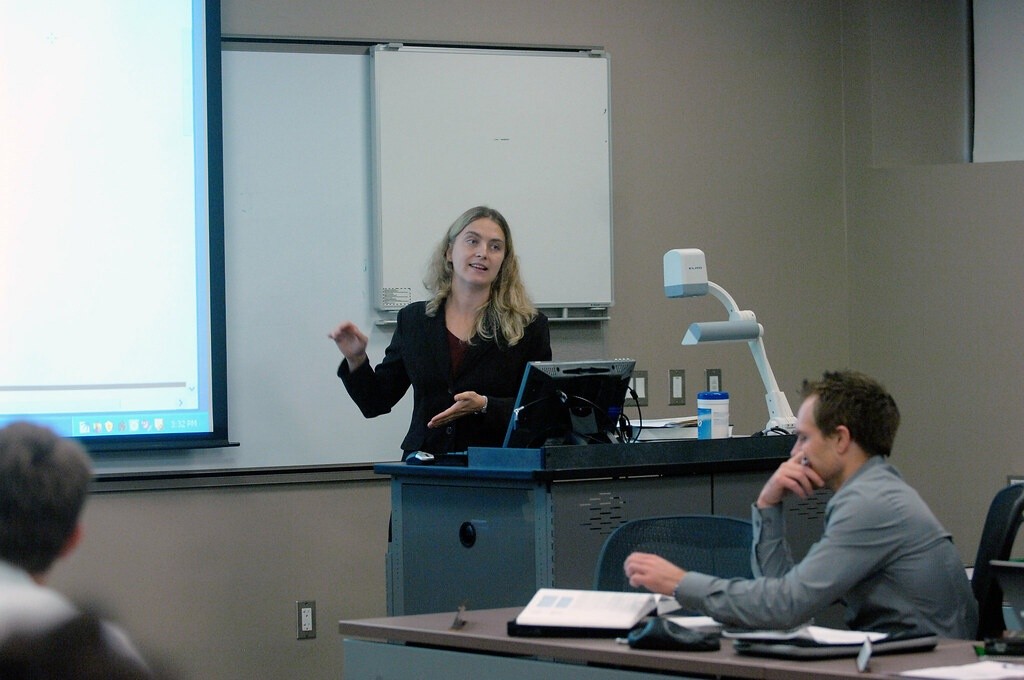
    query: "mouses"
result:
[406,451,435,465]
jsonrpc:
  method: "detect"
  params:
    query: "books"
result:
[629,425,733,441]
[722,625,937,659]
[509,587,682,638]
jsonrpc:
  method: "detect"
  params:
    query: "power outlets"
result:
[623,371,649,406]
[668,369,686,406]
[295,600,316,639]
[706,368,722,392]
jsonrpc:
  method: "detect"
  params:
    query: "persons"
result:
[0,421,155,680]
[623,369,980,639]
[328,207,553,464]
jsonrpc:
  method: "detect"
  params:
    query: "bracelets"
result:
[481,395,488,414]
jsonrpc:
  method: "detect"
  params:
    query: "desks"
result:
[338,606,1024,680]
[375,434,851,646]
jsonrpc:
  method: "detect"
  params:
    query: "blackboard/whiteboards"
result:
[95,36,616,474]
[369,42,611,326]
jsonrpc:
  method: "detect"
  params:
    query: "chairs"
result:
[964,482,1024,640]
[593,515,753,594]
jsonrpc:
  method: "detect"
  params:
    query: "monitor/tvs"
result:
[501,358,636,450]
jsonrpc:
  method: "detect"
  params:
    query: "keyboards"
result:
[438,451,469,464]
